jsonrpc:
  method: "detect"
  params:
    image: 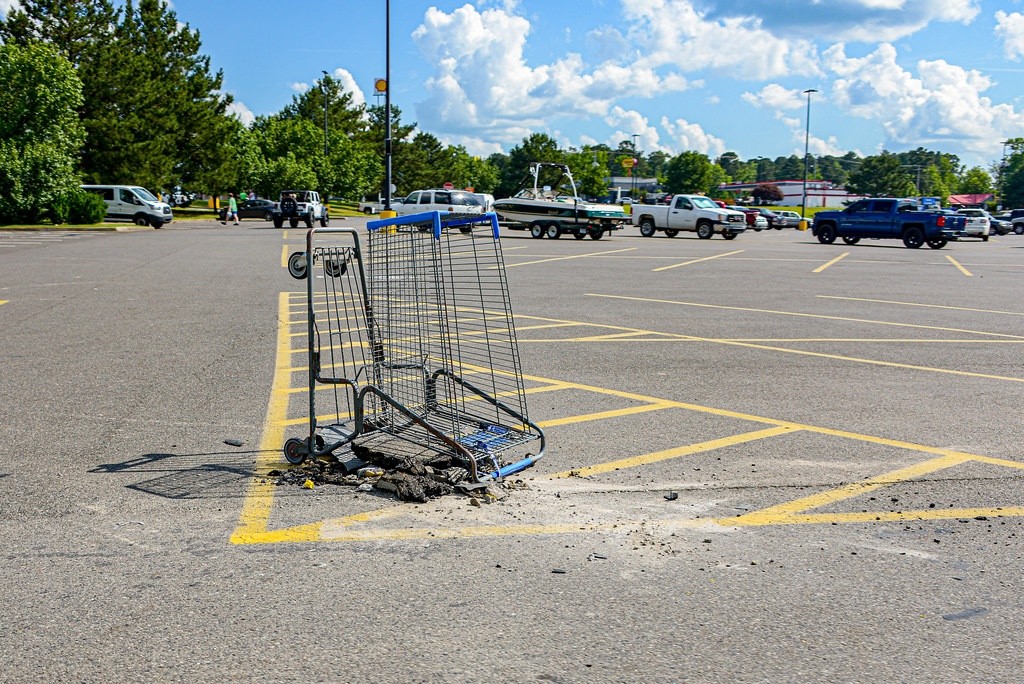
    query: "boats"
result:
[490,162,627,226]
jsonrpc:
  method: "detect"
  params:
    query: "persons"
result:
[156,190,163,202]
[238,189,257,201]
[221,193,239,226]
[127,192,133,203]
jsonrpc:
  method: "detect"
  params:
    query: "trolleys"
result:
[284,210,545,482]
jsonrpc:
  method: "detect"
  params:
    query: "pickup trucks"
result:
[626,195,747,240]
[358,198,394,214]
[812,197,967,249]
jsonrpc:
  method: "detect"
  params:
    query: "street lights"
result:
[798,90,818,231]
[322,70,330,204]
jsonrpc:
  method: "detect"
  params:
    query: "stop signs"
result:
[443,182,454,189]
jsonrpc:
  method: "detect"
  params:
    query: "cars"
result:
[219,199,281,221]
[147,186,196,207]
[748,207,787,230]
[773,211,814,230]
[988,215,1014,236]
[954,209,991,241]
[713,200,768,232]
[615,193,674,206]
[994,209,1024,235]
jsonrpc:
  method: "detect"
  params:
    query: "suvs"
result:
[273,190,329,229]
[390,188,483,234]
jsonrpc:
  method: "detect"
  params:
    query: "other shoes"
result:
[221,221,227,225]
[233,223,239,225]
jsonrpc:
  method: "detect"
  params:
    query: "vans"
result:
[79,184,173,229]
